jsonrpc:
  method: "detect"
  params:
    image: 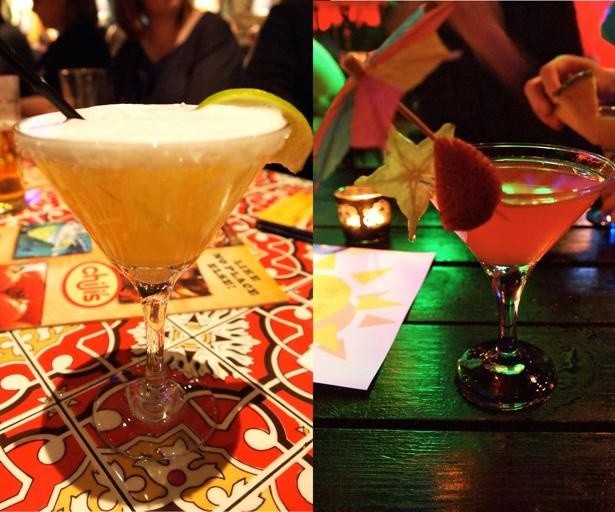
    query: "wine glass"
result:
[430,143,614,413]
[14,103,292,459]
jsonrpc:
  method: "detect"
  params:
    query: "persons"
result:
[520,53,615,154]
[15,0,106,95]
[409,1,607,158]
[97,1,246,108]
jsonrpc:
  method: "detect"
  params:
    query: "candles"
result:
[335,186,392,244]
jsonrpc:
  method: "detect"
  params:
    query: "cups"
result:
[1,76,22,201]
[59,66,109,106]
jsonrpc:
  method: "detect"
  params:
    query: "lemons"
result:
[195,88,315,174]
